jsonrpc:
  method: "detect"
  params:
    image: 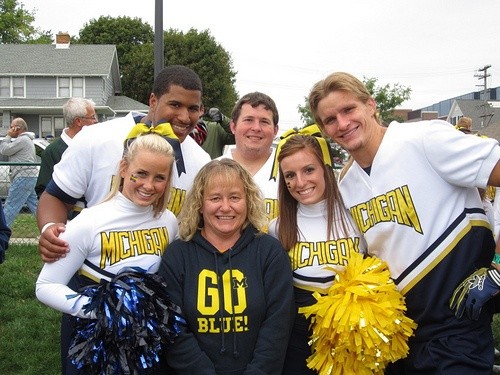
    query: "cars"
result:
[0,137,50,199]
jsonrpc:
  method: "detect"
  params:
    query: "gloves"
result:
[448,267,500,320]
[189,119,209,146]
[209,108,224,124]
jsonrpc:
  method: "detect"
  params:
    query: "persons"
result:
[37,65,212,375]
[35,134,180,319]
[309,72,500,375]
[0,117,38,227]
[189,100,235,160]
[158,158,295,375]
[34,97,98,220]
[267,123,367,375]
[212,91,281,231]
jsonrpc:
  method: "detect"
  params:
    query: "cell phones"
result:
[17,127,20,131]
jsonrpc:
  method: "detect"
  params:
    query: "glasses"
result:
[78,113,98,121]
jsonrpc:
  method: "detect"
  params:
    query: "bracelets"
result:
[41,222,55,235]
